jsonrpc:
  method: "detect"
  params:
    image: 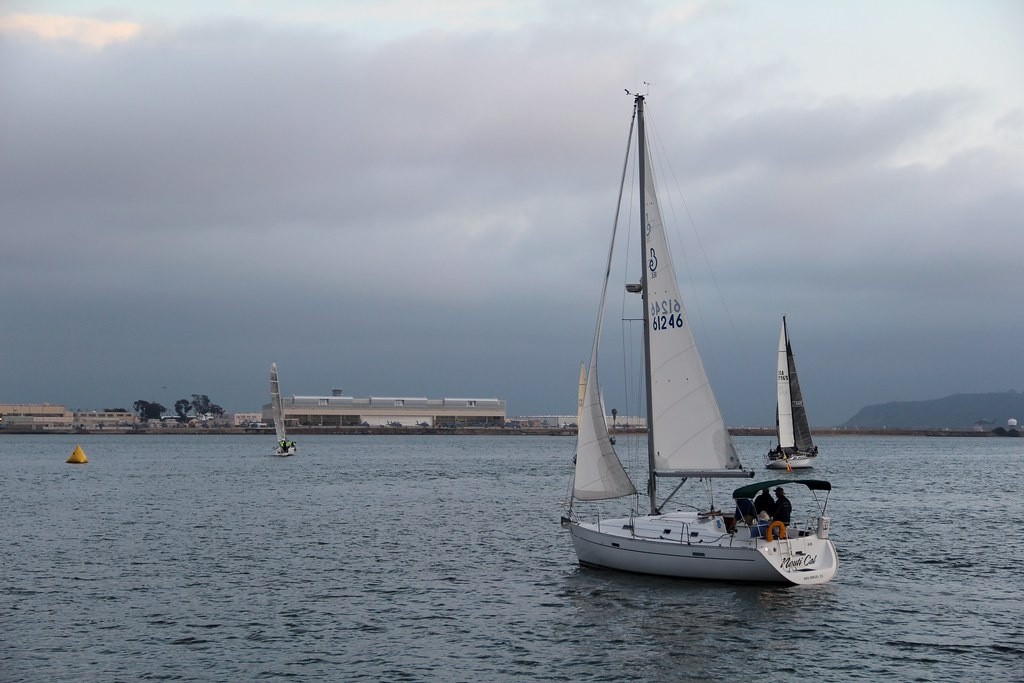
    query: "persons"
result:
[730,488,793,539]
[278,439,296,452]
[806,445,818,456]
[726,457,737,470]
[768,444,799,461]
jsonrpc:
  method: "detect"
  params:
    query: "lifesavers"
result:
[766,521,786,542]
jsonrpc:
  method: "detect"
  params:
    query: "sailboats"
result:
[558,94,838,589]
[760,313,821,470]
[268,362,297,455]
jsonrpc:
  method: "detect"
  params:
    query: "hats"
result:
[774,487,786,494]
